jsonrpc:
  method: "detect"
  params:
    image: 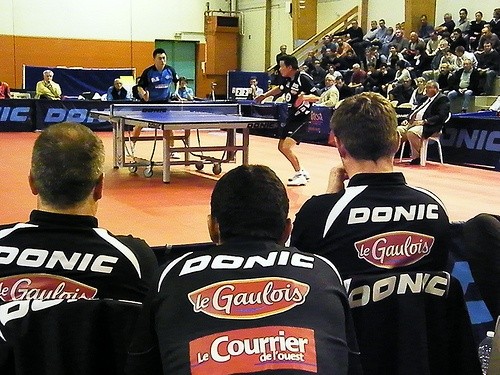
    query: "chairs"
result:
[398,111,451,168]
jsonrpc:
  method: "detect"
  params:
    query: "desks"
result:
[90,109,278,183]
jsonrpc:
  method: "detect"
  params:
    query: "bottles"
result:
[478,330,495,375]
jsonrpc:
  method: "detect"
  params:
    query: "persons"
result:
[271,6,500,113]
[0,120,164,375]
[33,69,62,100]
[254,55,323,188]
[288,91,454,280]
[250,76,264,100]
[155,164,363,374]
[178,77,194,101]
[129,48,183,160]
[394,80,450,166]
[106,78,128,102]
[0,81,12,99]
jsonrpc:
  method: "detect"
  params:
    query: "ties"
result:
[409,99,432,125]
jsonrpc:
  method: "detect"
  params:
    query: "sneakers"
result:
[286,171,310,186]
[460,110,466,113]
[409,157,420,165]
[125,141,136,158]
[168,146,181,159]
[480,91,489,95]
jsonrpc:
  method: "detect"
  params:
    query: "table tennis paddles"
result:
[293,95,304,108]
[143,90,150,101]
